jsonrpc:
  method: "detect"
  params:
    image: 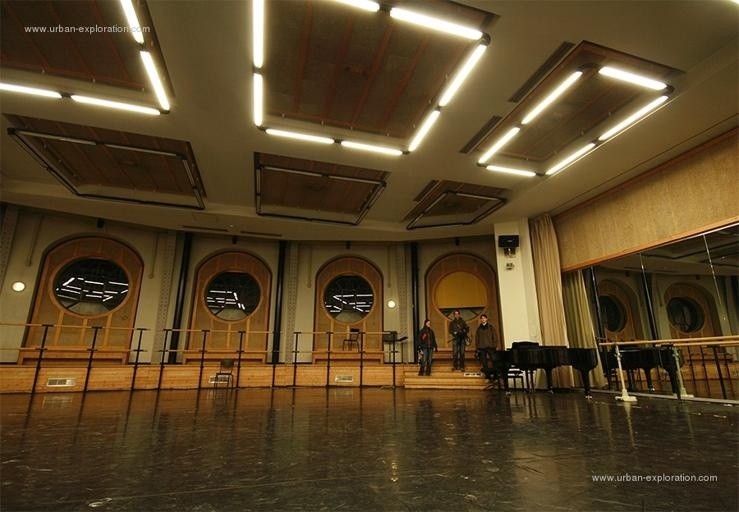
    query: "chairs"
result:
[342,329,360,354]
[212,358,235,401]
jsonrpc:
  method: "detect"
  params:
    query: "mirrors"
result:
[561,215,739,405]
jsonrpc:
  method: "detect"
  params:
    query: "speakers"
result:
[498,235,519,247]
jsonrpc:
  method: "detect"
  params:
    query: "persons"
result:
[474,314,499,384]
[448,309,469,372]
[416,319,438,376]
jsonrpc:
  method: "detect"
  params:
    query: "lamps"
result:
[252,0,490,157]
[56,276,129,302]
[6,127,206,211]
[1,1,171,116]
[325,294,374,313]
[254,165,387,227]
[206,290,246,310]
[407,189,507,231]
[475,62,675,178]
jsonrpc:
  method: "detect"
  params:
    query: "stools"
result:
[497,373,524,392]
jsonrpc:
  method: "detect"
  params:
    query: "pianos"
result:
[498,342,598,399]
[600,339,684,393]
